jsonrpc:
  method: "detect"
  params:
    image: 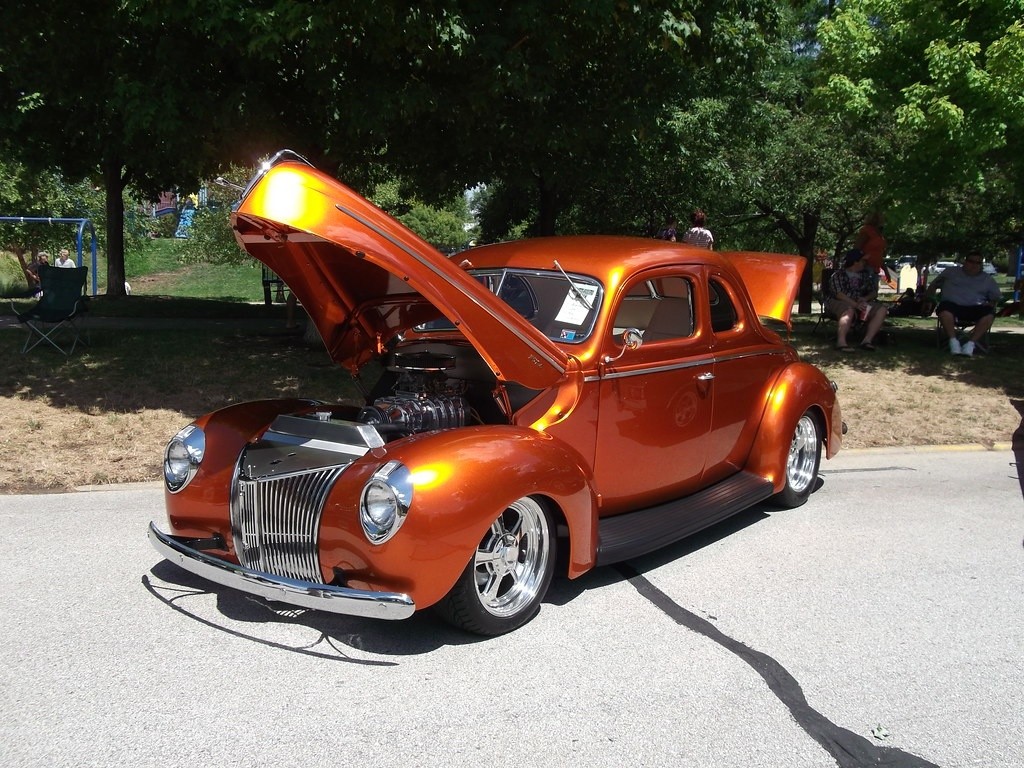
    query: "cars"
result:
[982,262,997,276]
[921,261,957,273]
[146,147,848,638]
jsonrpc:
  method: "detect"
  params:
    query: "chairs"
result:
[809,269,865,345]
[16,265,90,358]
[937,317,991,354]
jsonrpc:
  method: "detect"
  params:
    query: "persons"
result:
[55,249,76,268]
[853,206,891,293]
[927,251,1001,357]
[887,288,915,317]
[286,293,301,328]
[681,210,714,251]
[26,252,50,297]
[658,215,678,242]
[824,248,887,352]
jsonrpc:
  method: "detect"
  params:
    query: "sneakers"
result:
[950,338,975,357]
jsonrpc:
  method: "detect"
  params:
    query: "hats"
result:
[844,249,864,268]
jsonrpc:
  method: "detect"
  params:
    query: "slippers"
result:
[837,345,856,353]
[860,343,875,351]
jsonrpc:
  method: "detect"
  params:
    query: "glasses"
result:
[965,259,983,264]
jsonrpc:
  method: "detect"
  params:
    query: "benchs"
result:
[613,298,690,352]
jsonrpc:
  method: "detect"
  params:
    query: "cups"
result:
[860,305,872,320]
